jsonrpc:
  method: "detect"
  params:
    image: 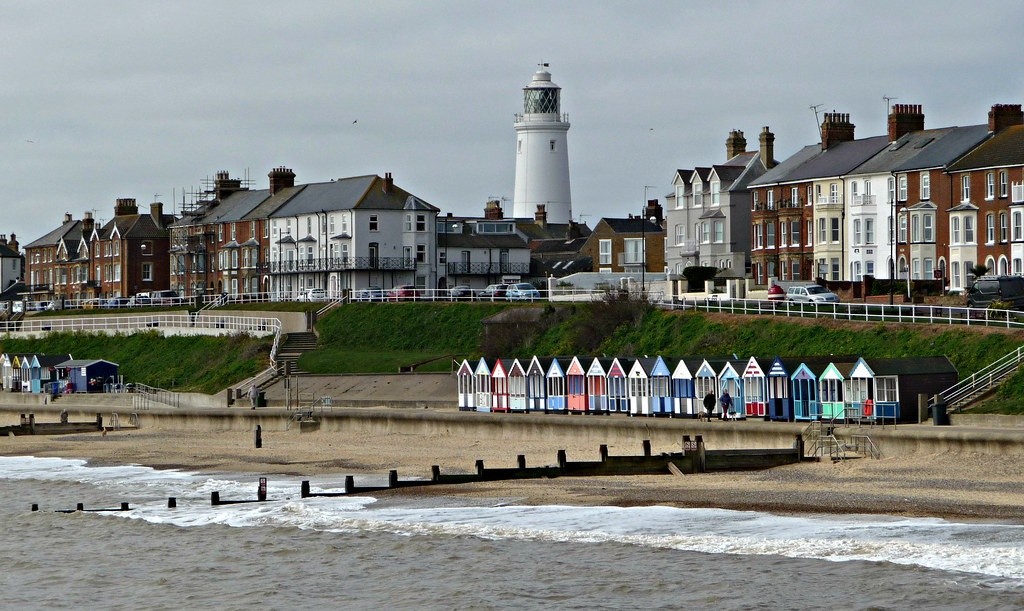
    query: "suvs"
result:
[478,284,510,301]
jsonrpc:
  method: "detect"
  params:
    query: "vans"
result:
[963,273,1024,311]
[786,284,840,307]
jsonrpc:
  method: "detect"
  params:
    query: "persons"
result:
[720,388,733,421]
[247,384,259,410]
[703,389,716,422]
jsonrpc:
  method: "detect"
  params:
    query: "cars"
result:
[10,301,51,313]
[386,285,421,301]
[296,288,326,302]
[356,286,387,301]
[151,290,183,306]
[448,286,477,302]
[505,283,540,301]
[102,297,129,310]
[127,295,151,307]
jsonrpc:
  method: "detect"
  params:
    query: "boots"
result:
[723,416,728,421]
[708,415,711,422]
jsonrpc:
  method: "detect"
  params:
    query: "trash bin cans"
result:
[932,403,947,426]
[49,382,59,394]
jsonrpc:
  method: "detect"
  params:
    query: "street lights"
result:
[445,216,459,301]
[890,204,907,307]
[279,232,290,302]
[135,244,147,309]
[643,214,657,301]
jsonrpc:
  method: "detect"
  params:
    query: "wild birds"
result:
[648,127,654,132]
[352,119,358,124]
